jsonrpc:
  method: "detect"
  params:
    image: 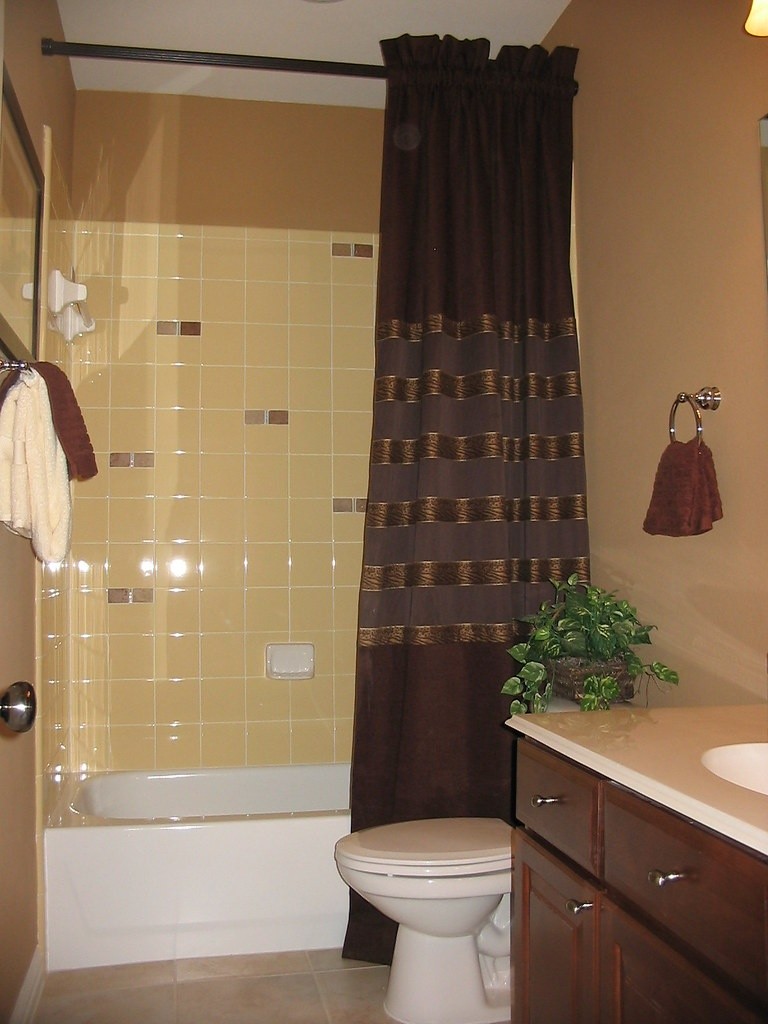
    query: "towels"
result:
[1,361,100,567]
[641,435,724,537]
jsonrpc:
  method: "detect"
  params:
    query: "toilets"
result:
[333,689,642,1022]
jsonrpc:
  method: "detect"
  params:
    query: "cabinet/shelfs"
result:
[511,736,768,1024]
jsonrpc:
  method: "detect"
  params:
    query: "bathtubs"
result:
[42,763,353,969]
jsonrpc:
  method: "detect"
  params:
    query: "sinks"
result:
[700,742,768,795]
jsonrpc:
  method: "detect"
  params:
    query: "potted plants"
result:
[501,573,680,716]
